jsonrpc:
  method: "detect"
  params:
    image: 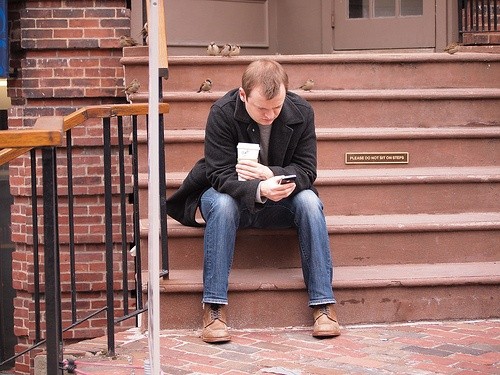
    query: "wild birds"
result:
[298,79,314,92]
[443,44,458,54]
[196,78,214,93]
[118,35,138,48]
[120,79,141,95]
[220,44,241,57]
[207,42,220,56]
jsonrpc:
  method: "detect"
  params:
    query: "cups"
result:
[236,142,260,181]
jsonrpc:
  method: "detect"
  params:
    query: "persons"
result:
[165,59,341,343]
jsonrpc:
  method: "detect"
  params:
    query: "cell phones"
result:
[279,175,297,184]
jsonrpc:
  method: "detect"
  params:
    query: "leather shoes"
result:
[203,307,230,342]
[313,303,341,336]
